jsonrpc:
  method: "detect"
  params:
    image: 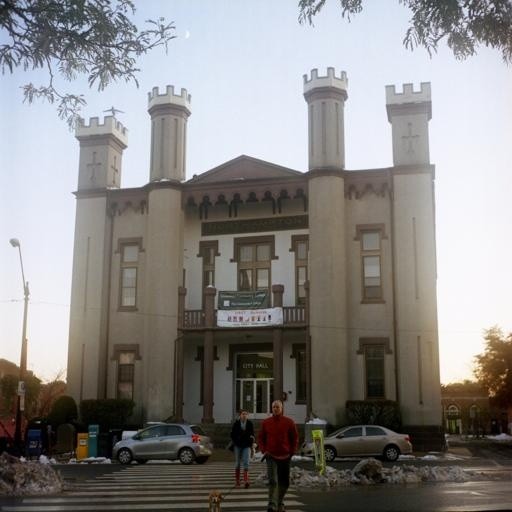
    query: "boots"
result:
[234,469,250,487]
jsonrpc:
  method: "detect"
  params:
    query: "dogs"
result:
[208,490,223,512]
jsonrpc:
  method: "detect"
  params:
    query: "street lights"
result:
[8,238,29,441]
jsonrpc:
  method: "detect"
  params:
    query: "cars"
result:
[112,422,214,466]
[300,424,413,462]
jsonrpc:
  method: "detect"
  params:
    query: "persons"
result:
[229,408,255,488]
[257,399,300,512]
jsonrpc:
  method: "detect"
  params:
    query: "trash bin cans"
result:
[26,418,49,460]
[305,423,326,442]
